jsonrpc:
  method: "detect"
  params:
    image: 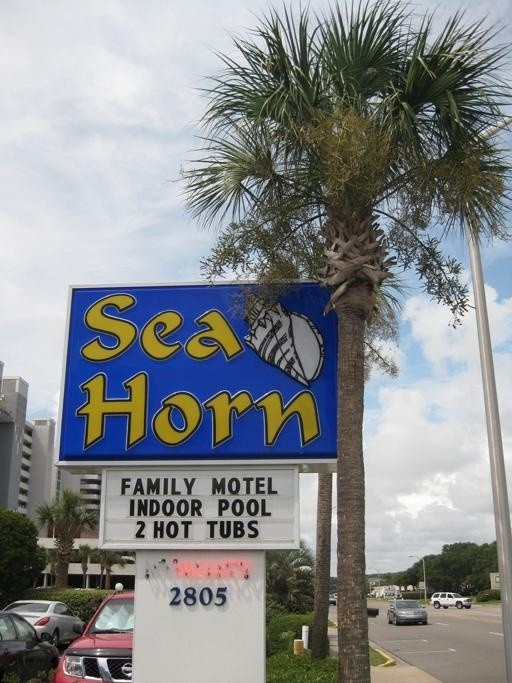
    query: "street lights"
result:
[408,555,427,602]
[366,566,381,600]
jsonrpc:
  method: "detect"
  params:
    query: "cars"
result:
[387,600,428,625]
[0,610,61,682]
[328,592,337,605]
[2,599,83,649]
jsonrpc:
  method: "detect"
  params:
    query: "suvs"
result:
[54,590,136,682]
[431,592,473,609]
